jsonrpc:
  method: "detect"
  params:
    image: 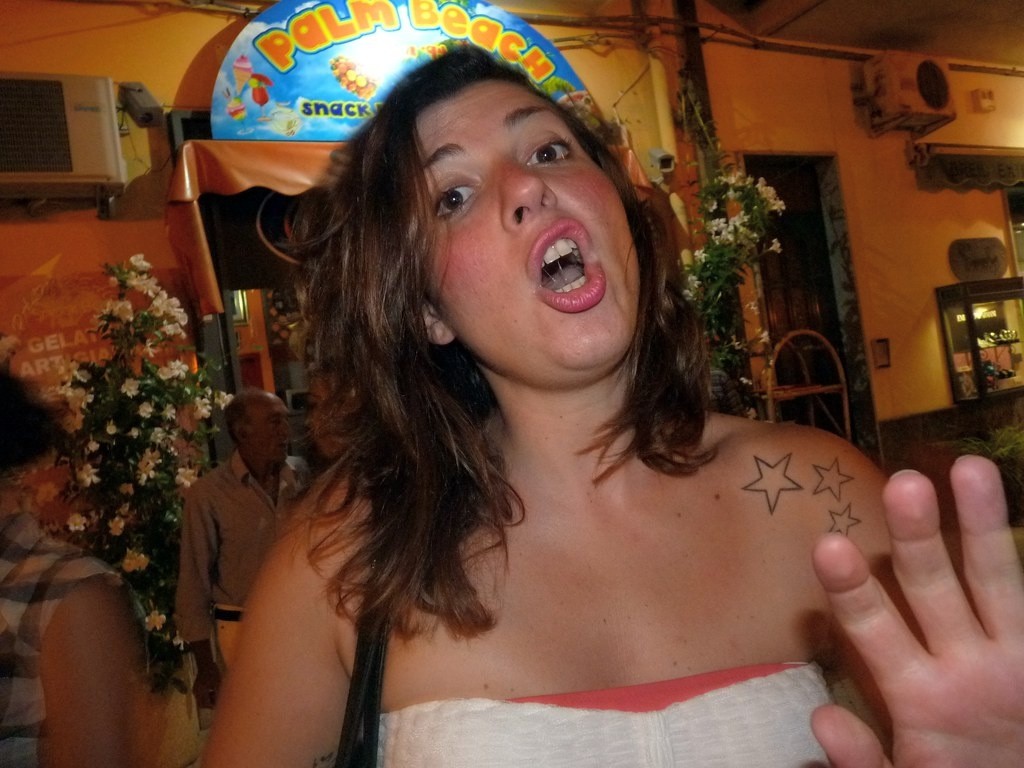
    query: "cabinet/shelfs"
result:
[934,277,1024,410]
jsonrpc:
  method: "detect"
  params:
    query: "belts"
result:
[212,607,243,622]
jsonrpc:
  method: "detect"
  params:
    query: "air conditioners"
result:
[864,54,958,129]
[0,71,127,202]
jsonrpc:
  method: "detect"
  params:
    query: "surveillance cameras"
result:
[649,148,674,172]
[117,81,164,128]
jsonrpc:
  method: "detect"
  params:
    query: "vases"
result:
[139,648,201,768]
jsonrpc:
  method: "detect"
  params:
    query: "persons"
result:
[175,389,313,709]
[189,45,1024,768]
[0,366,150,768]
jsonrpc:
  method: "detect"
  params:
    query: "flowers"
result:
[679,89,786,422]
[50,251,235,648]
